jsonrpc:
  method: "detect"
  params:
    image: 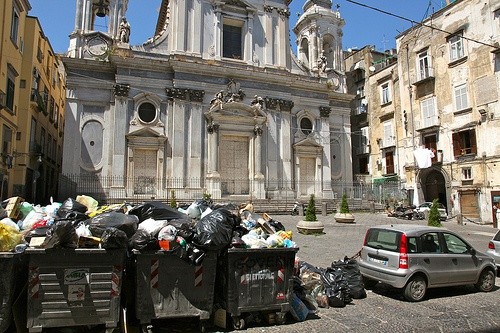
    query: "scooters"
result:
[291,201,308,215]
[395,202,413,220]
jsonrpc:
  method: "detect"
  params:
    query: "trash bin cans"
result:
[225,204,300,330]
[0,195,24,333]
[25,195,137,333]
[135,199,240,333]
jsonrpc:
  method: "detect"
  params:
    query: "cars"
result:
[359,223,498,302]
[487,228,500,276]
[413,202,448,221]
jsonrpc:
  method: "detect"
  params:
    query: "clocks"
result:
[83,32,110,60]
